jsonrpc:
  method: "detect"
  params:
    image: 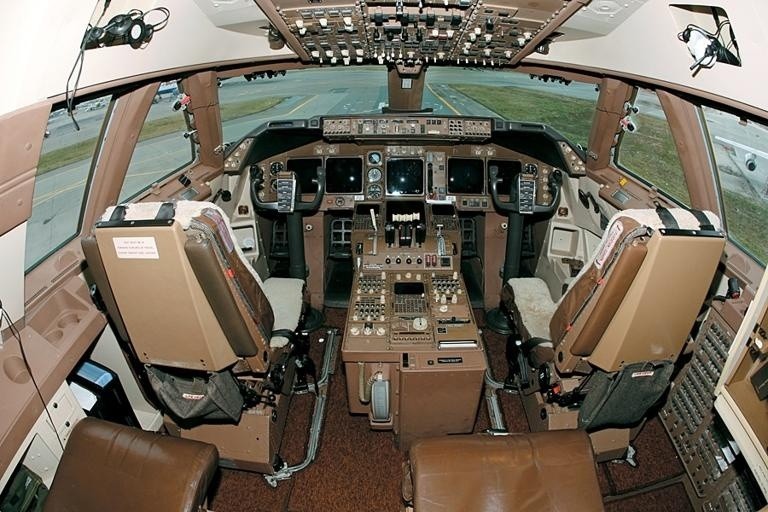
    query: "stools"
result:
[396,422,606,512]
[38,413,226,512]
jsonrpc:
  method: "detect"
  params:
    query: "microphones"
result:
[690,46,713,70]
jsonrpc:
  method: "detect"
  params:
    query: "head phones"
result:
[81,7,170,50]
[683,20,742,71]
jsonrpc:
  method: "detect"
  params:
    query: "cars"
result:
[45,81,179,120]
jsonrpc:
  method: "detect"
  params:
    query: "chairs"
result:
[76,195,318,479]
[490,200,728,463]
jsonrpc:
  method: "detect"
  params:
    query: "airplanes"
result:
[711,136,768,203]
